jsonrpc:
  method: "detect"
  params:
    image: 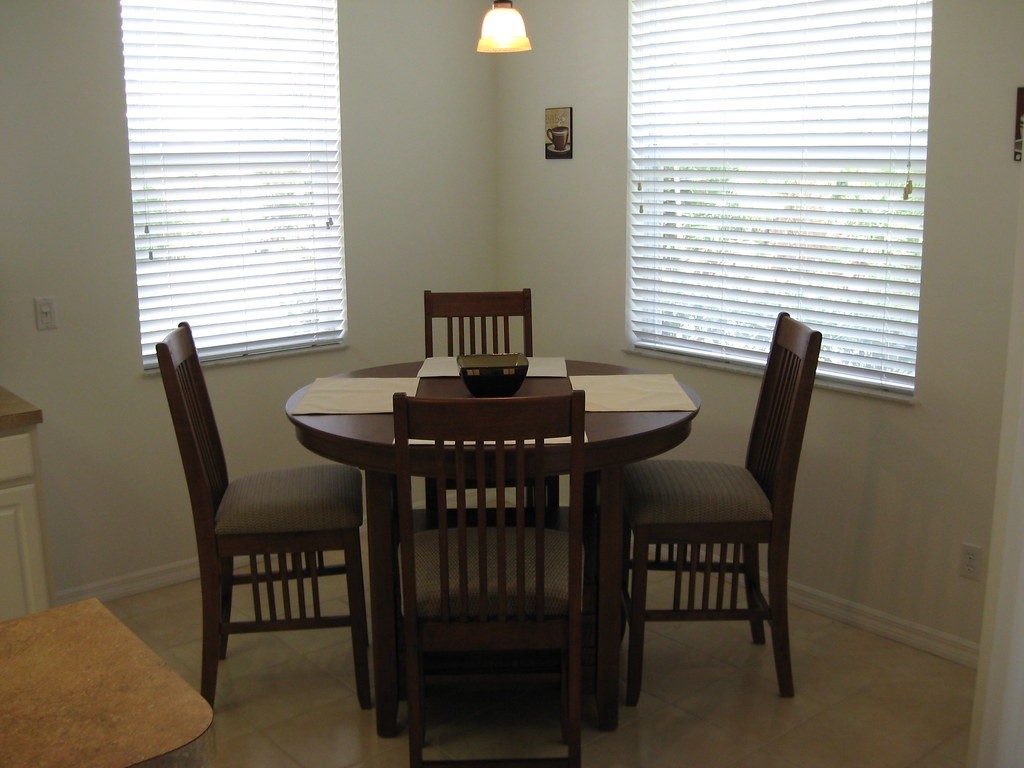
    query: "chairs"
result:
[391,389,588,768]
[624,312,823,708]
[423,287,561,511]
[156,321,373,710]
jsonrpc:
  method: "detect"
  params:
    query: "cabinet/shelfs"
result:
[0,382,54,623]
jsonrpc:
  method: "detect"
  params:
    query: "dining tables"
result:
[286,358,702,739]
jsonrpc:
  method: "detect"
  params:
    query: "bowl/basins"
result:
[456,352,528,398]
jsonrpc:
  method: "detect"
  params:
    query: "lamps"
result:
[476,0,532,54]
